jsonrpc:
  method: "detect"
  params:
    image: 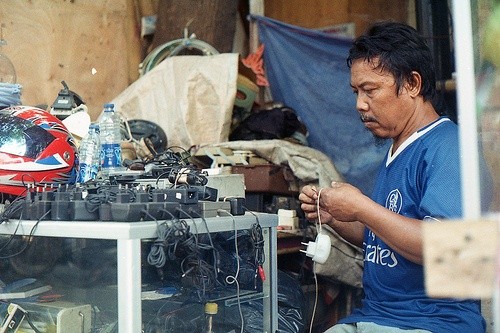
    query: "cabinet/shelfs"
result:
[0,211,278,332]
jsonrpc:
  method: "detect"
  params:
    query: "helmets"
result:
[0,105,78,198]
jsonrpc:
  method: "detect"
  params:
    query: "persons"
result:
[299,21,488,332]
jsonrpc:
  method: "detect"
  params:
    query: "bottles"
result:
[99,104,123,175]
[76,123,100,185]
